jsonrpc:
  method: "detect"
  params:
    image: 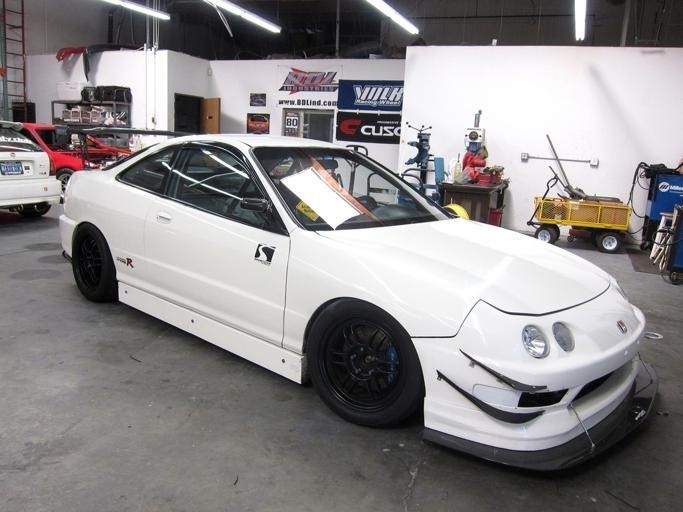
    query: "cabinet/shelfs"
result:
[50,100,131,150]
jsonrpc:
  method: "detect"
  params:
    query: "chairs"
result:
[209,164,264,226]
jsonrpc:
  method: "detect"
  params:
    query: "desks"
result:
[442,180,509,225]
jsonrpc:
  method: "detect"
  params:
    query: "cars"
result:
[58,132,659,473]
[0,121,135,217]
